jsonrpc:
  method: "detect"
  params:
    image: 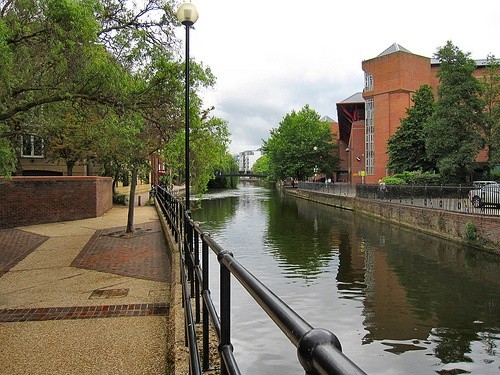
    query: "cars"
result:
[468,184,500,208]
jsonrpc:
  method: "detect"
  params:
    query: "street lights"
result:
[176,4,199,211]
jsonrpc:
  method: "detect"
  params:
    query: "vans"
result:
[473,181,500,194]
